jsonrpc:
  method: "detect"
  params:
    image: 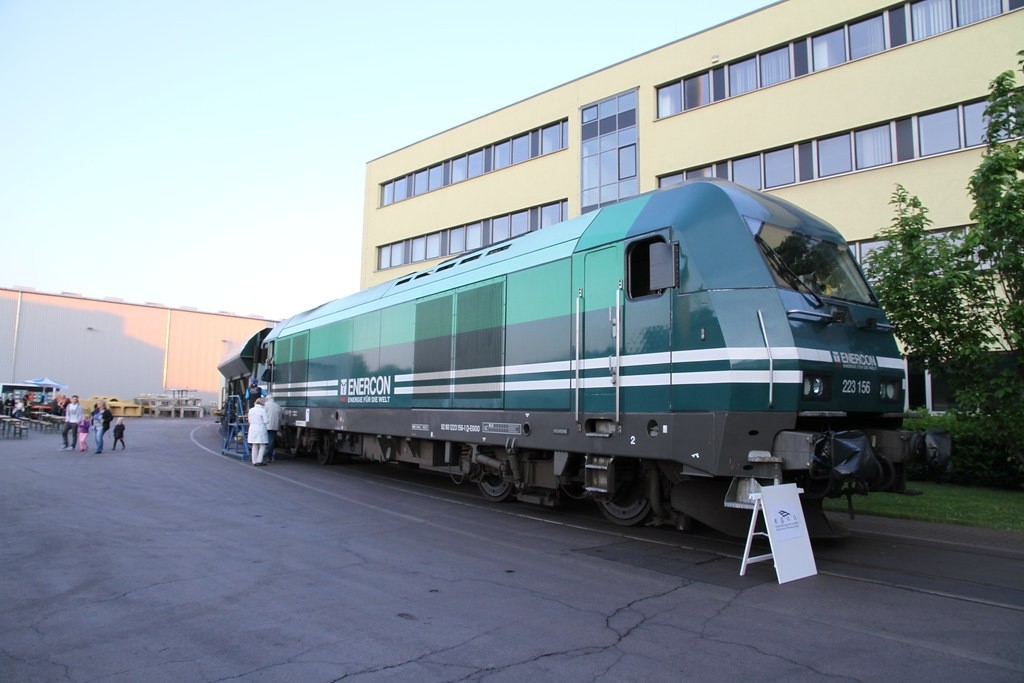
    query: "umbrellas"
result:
[20,377,68,394]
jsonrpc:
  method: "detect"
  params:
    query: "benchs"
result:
[14,417,53,440]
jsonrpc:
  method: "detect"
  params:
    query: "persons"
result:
[0,393,34,418]
[111,417,126,450]
[248,395,282,466]
[91,400,114,454]
[77,414,90,451]
[48,393,71,416]
[61,395,82,451]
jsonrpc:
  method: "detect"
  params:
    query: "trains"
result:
[258,175,955,548]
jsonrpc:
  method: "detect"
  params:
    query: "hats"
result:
[8,393,13,398]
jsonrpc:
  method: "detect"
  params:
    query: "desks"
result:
[30,412,46,429]
[2,419,21,440]
[51,416,66,432]
[0,415,11,434]
[41,415,56,430]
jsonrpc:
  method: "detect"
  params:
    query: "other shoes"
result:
[80,449,84,452]
[84,447,87,451]
[255,463,267,466]
[122,447,125,450]
[112,448,115,450]
[65,446,74,450]
[95,452,101,454]
[56,445,67,451]
